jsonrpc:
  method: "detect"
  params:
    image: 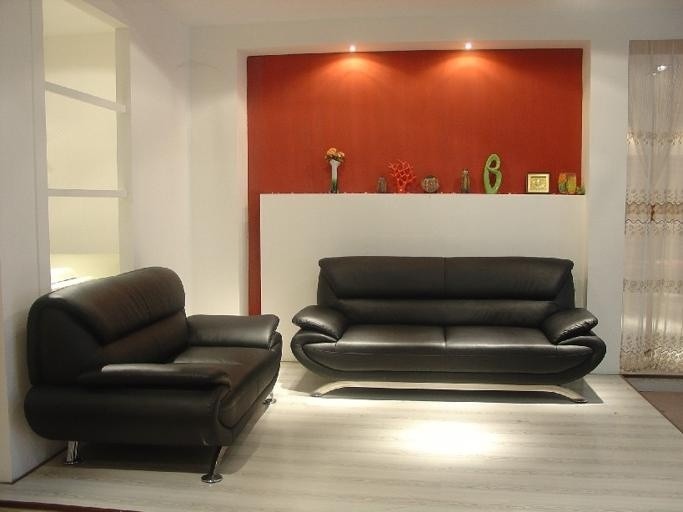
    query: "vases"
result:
[329,159,342,193]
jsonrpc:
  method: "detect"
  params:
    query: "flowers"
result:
[323,147,345,163]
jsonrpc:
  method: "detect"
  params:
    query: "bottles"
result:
[461,168,471,193]
[376,176,386,193]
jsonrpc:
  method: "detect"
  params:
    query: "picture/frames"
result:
[525,171,552,194]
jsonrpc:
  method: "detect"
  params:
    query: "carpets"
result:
[1,362,682,511]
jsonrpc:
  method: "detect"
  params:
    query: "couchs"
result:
[24,266,284,485]
[291,255,608,404]
